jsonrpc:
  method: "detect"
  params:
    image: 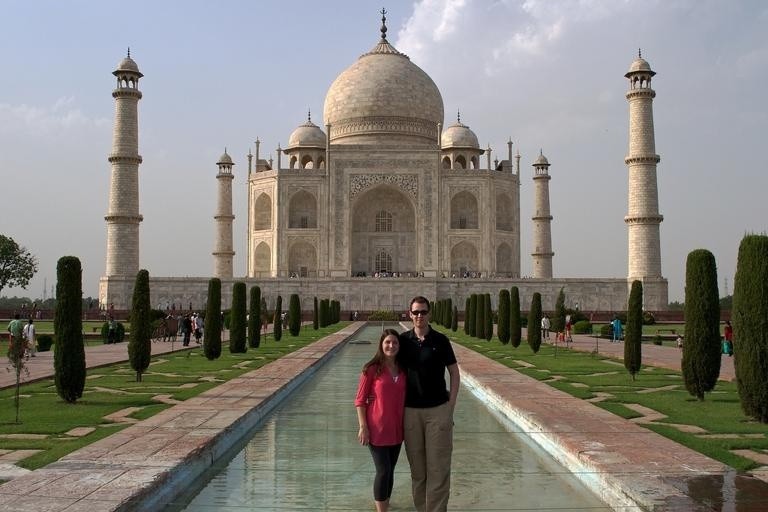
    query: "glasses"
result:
[411,310,430,315]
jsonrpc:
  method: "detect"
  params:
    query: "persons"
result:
[575,303,578,311]
[590,311,593,321]
[541,314,551,340]
[351,270,517,278]
[724,320,734,356]
[81,302,114,323]
[608,317,622,343]
[559,314,573,343]
[354,310,358,320]
[355,329,409,512]
[352,311,355,320]
[677,335,683,352]
[396,297,459,511]
[157,302,288,346]
[4,301,42,358]
[288,271,299,278]
